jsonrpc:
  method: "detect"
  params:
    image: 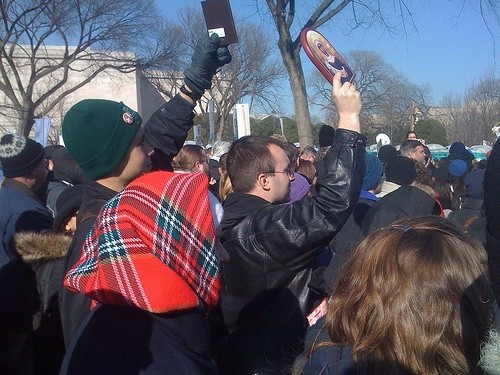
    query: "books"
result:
[201,0,238,45]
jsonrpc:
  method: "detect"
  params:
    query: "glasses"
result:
[263,166,295,179]
[200,157,210,164]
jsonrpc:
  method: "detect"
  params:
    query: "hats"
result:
[448,159,468,175]
[463,169,485,196]
[0,134,45,178]
[51,187,82,231]
[448,142,466,155]
[360,152,384,191]
[377,144,397,162]
[384,156,417,185]
[207,190,224,232]
[60,97,143,181]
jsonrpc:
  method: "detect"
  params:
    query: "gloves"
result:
[182,33,234,90]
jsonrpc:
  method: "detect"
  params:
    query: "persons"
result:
[314,39,353,85]
[0,32,500,375]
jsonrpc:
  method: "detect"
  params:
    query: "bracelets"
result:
[179,86,202,102]
